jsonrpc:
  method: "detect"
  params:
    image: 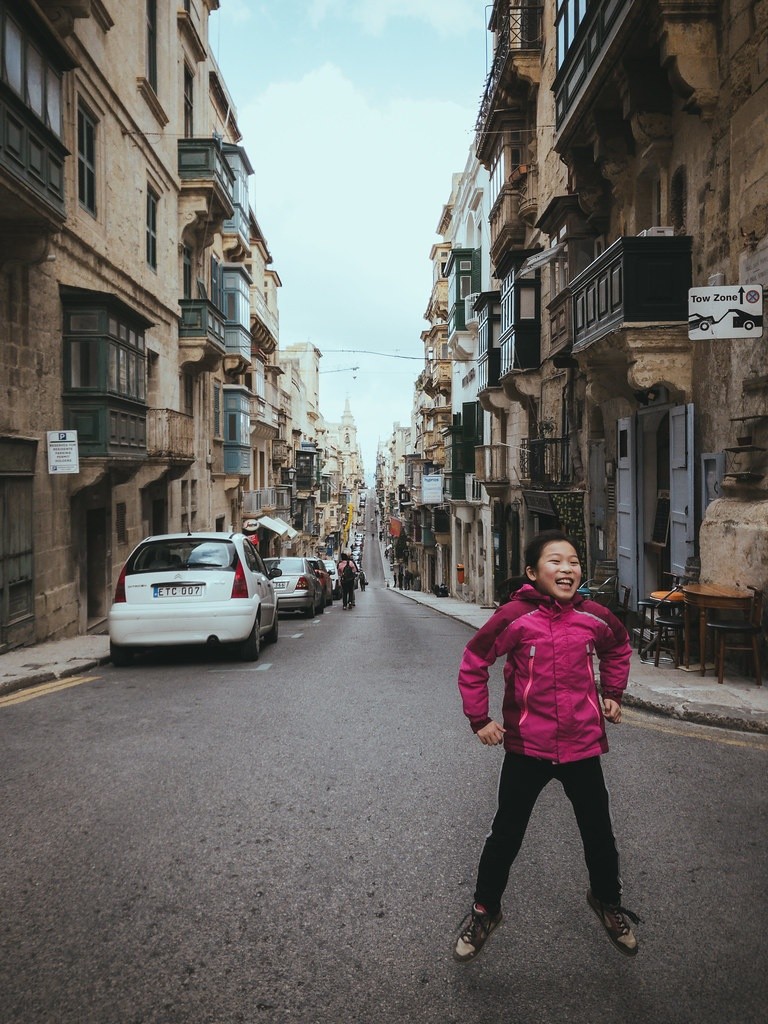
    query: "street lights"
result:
[289,465,296,528]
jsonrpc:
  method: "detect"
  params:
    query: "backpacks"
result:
[342,560,355,582]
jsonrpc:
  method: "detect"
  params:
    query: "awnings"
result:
[257,516,298,541]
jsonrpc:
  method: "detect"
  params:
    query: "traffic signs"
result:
[688,285,764,340]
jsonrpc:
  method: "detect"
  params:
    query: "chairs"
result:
[653,575,701,671]
[608,585,632,630]
[588,574,619,609]
[636,571,685,660]
[705,585,768,688]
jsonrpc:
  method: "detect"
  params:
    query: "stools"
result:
[639,590,685,662]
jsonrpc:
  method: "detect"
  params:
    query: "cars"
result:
[261,488,384,618]
[109,531,280,666]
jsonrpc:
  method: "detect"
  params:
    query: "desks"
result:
[679,583,756,678]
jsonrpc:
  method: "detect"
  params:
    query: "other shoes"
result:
[343,602,355,610]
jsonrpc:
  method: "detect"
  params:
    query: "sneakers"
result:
[454,902,504,962]
[586,889,639,956]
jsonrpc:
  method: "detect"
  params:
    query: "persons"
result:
[392,569,413,590]
[337,554,359,606]
[450,529,646,975]
[337,553,357,611]
[358,568,366,592]
